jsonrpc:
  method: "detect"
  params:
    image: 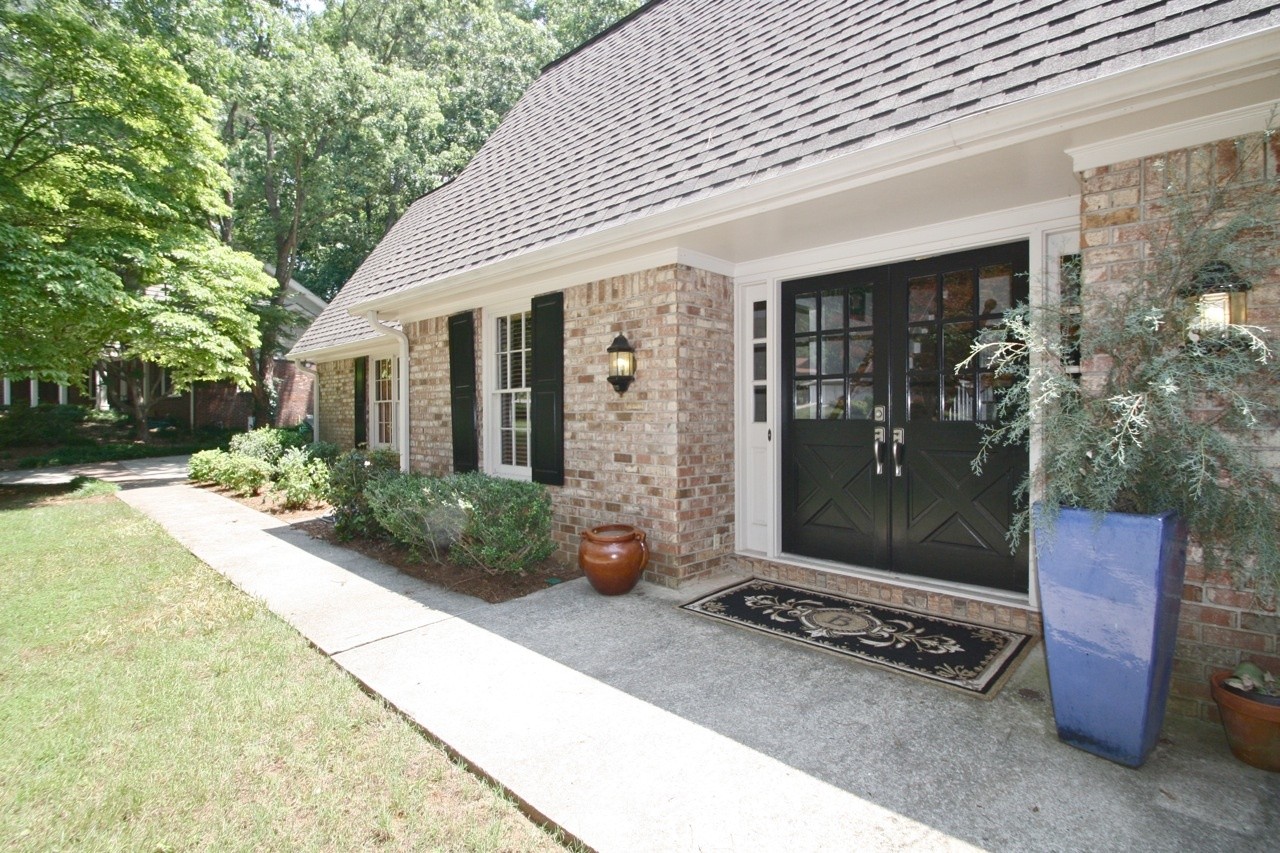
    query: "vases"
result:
[1211,672,1280,773]
[579,523,650,594]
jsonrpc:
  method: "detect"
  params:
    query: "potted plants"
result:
[953,100,1280,768]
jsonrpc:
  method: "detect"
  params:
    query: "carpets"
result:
[677,575,1041,704]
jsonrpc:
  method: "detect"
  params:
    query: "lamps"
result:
[606,332,637,396]
[1178,259,1253,353]
